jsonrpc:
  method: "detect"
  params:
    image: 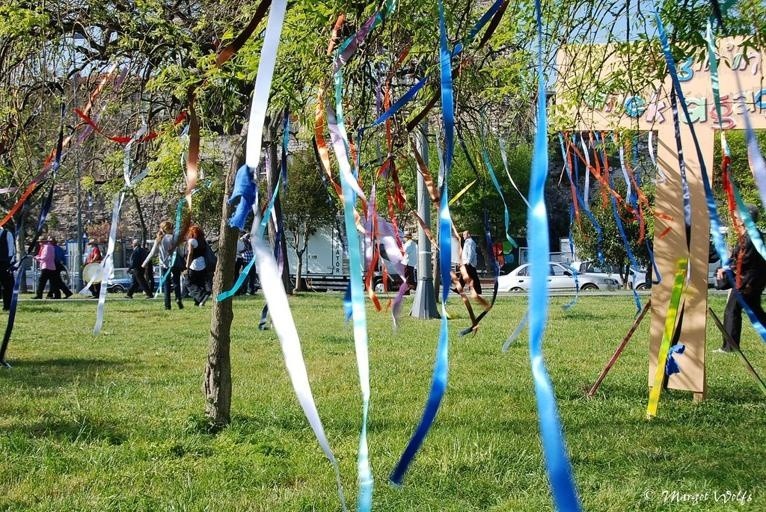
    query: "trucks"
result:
[262,212,412,294]
[708,226,731,285]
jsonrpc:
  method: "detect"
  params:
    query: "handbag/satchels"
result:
[205,244,217,272]
[713,267,736,290]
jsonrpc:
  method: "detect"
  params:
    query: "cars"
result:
[492,259,623,293]
[571,259,649,291]
[88,265,174,295]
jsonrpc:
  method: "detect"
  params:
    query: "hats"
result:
[88,239,98,245]
[47,236,54,241]
[403,231,413,237]
[36,236,47,242]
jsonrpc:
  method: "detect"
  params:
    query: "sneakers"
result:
[176,299,184,309]
[46,296,61,299]
[30,296,42,299]
[199,295,208,306]
[63,292,73,299]
[450,288,459,294]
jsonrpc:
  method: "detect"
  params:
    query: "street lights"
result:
[73,152,108,294]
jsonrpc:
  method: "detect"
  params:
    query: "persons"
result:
[450,230,482,294]
[30,221,256,309]
[402,231,418,295]
[712,204,766,355]
[0,228,15,310]
